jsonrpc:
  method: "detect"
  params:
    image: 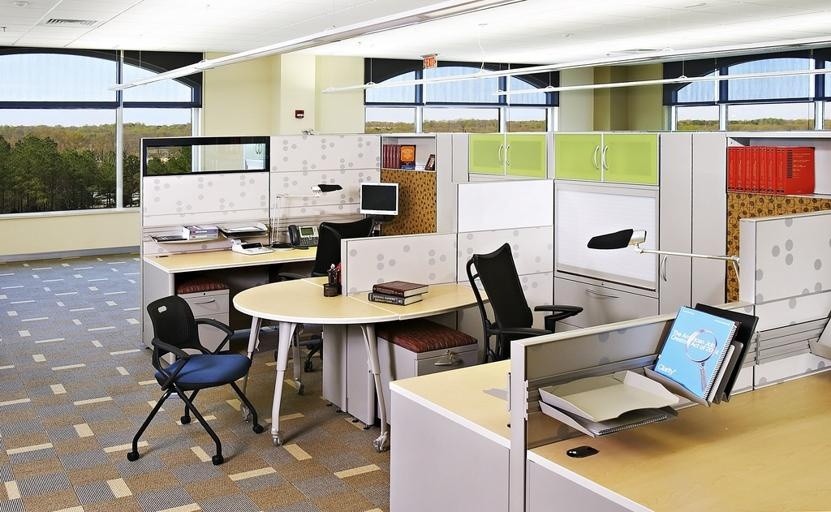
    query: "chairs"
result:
[467,243,583,364]
[127,296,264,464]
[275,217,377,371]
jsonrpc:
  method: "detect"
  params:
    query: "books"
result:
[373,281,429,298]
[383,144,416,170]
[652,303,760,404]
[727,146,815,195]
[186,225,218,240]
[564,409,668,436]
[368,293,424,306]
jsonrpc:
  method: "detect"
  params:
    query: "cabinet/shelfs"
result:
[171,278,229,354]
[466,134,548,177]
[551,269,658,336]
[554,130,660,185]
[727,131,828,300]
[379,134,436,234]
[436,133,469,184]
[378,318,477,425]
[658,131,729,318]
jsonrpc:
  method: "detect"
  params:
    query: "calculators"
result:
[231,239,274,255]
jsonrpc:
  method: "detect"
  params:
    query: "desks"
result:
[527,369,831,512]
[388,358,515,511]
[142,230,318,365]
[231,265,491,450]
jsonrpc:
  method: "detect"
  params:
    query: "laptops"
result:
[360,185,398,235]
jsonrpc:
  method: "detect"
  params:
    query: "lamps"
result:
[587,229,741,288]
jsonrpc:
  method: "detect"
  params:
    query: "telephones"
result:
[288,224,319,249]
